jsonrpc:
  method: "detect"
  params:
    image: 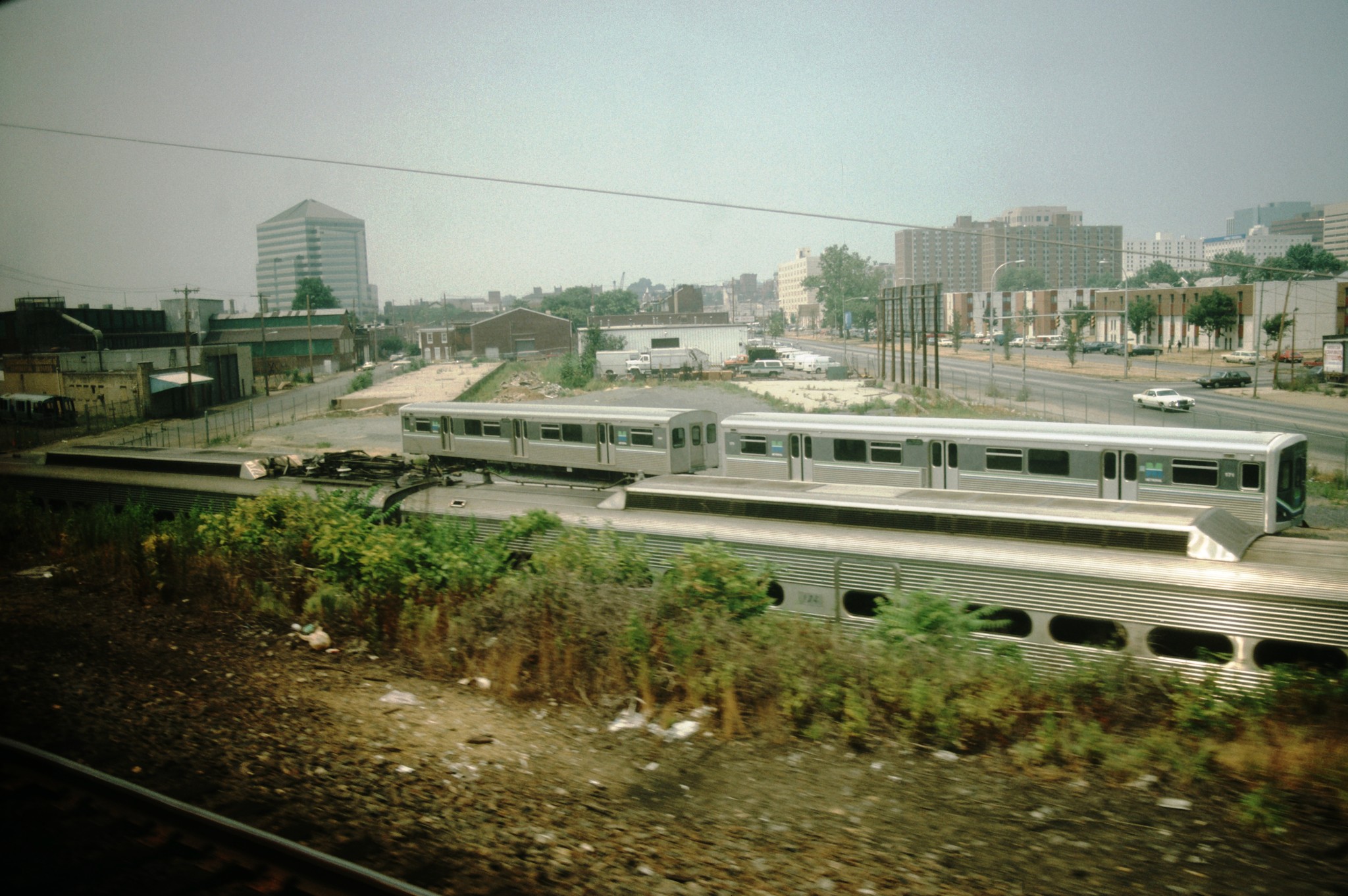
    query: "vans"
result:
[752,338,839,373]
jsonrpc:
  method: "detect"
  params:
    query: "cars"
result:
[362,362,375,370]
[1221,350,1267,365]
[1132,388,1196,412]
[1272,349,1304,363]
[389,352,404,362]
[926,337,936,345]
[937,338,954,347]
[721,355,744,368]
[1303,357,1324,367]
[957,329,1163,357]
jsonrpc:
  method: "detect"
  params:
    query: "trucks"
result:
[624,346,712,376]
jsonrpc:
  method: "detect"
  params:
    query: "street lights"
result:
[989,260,1026,378]
[1098,259,1129,379]
[842,296,869,363]
[819,285,837,330]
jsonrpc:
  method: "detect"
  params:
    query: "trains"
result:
[0,393,76,429]
[398,400,1310,535]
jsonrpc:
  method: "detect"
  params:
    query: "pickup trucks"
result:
[723,354,749,369]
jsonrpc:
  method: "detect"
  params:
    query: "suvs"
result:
[1194,370,1253,389]
[738,359,785,379]
